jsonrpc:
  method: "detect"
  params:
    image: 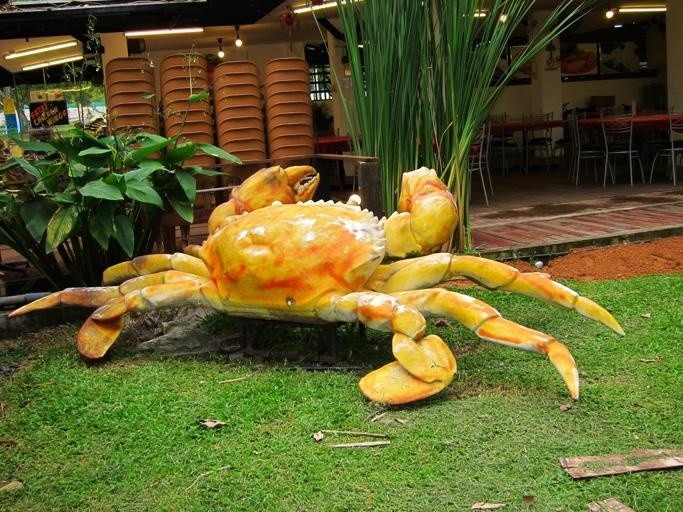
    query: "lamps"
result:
[470,111,683,207]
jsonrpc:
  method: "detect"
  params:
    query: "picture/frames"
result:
[315,136,363,191]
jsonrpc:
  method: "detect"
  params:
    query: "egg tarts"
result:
[6,164,625,405]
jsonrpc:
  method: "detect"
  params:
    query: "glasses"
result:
[217,24,243,58]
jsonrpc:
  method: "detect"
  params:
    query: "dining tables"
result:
[205,53,222,83]
